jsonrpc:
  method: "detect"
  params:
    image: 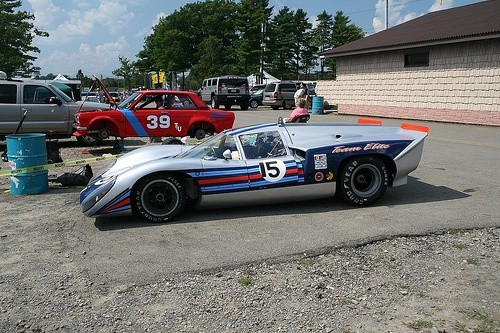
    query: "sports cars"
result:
[79,122,431,222]
[72,89,236,146]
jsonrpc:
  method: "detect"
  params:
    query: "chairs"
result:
[285,114,310,123]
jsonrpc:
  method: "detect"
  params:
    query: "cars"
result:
[249,84,266,108]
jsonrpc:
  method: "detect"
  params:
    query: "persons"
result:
[156,98,165,109]
[289,98,309,123]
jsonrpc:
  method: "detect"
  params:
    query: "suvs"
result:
[195,75,250,111]
[0,70,115,147]
[263,81,315,109]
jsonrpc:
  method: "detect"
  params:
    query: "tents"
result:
[247,66,281,84]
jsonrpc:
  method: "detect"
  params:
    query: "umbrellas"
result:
[36,81,71,94]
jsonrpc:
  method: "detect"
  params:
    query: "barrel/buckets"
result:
[5,133,49,195]
[311,96,324,114]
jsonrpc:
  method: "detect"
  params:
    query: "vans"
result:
[80,92,122,103]
